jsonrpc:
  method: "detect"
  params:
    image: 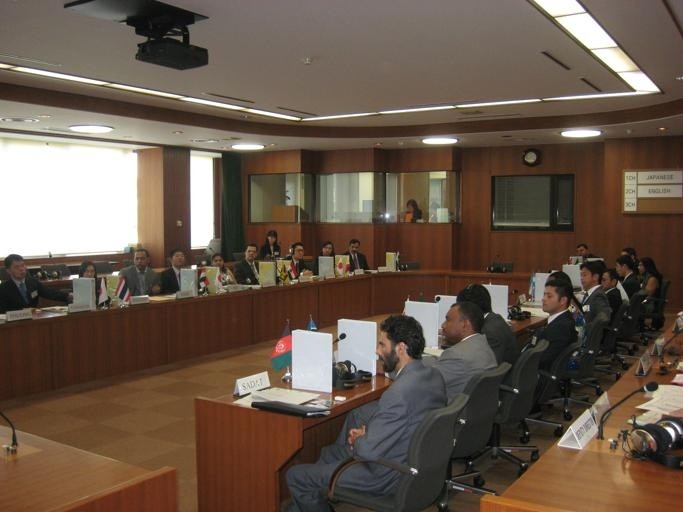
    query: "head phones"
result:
[507,305,531,321]
[624,415,683,469]
[400,263,409,271]
[33,270,62,280]
[288,242,297,253]
[333,361,371,389]
[485,265,507,273]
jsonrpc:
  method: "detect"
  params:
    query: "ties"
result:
[178,272,181,285]
[18,283,29,304]
[353,254,358,269]
[296,263,299,274]
[582,292,587,304]
[251,265,259,281]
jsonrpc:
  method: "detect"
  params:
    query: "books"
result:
[249,400,331,418]
[231,386,320,408]
[628,361,683,427]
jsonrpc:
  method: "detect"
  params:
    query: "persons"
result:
[120,249,185,295]
[285,311,448,512]
[212,231,280,284]
[400,197,422,222]
[336,303,497,443]
[456,283,522,376]
[79,259,99,283]
[0,255,73,316]
[288,238,371,278]
[531,244,661,376]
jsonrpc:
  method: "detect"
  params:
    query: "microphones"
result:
[596,381,658,439]
[434,297,440,303]
[333,333,346,345]
[0,411,18,448]
[508,289,518,296]
[345,359,357,372]
[656,328,683,375]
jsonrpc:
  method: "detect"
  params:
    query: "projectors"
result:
[136,37,209,70]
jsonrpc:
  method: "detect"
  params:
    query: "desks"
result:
[480,311,681,510]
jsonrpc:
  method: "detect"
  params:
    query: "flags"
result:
[99,280,108,306]
[199,268,222,288]
[115,278,132,302]
[278,267,289,281]
